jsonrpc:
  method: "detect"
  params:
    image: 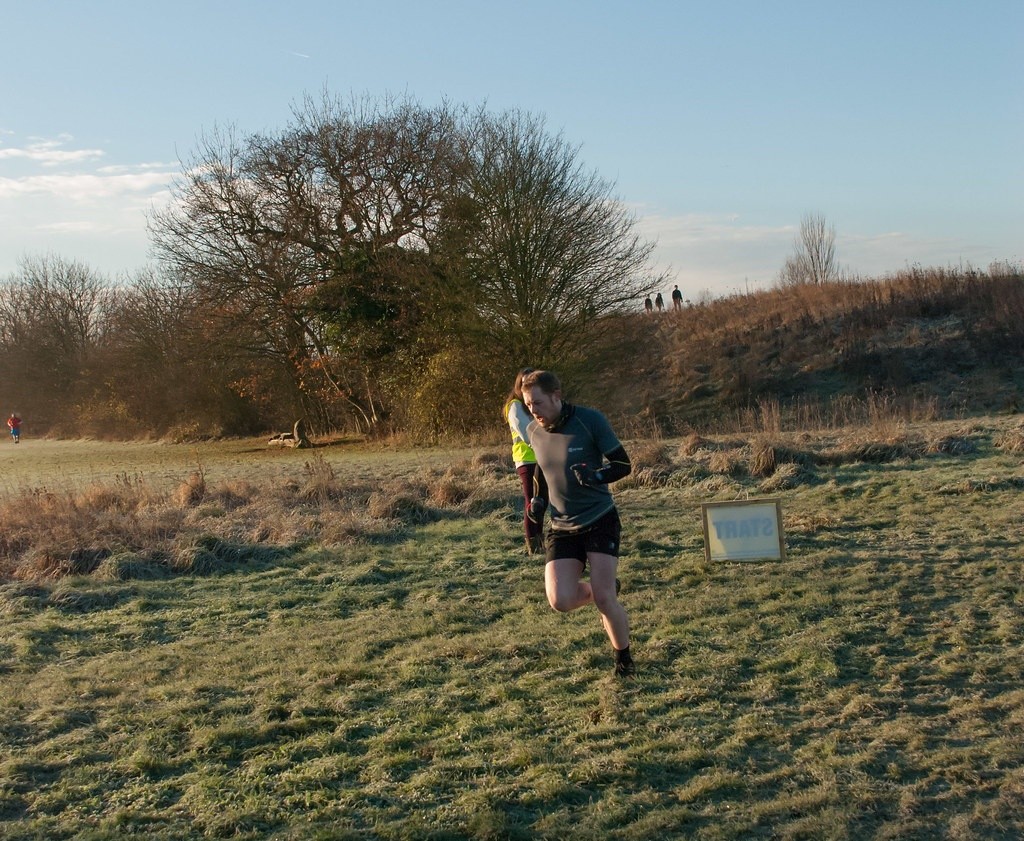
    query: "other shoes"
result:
[613,661,635,677]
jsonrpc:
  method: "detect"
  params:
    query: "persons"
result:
[7,413,23,443]
[503,367,548,561]
[655,293,664,311]
[520,371,635,677]
[672,285,683,312]
[645,294,652,313]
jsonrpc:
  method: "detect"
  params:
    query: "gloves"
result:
[526,497,545,523]
[570,463,598,486]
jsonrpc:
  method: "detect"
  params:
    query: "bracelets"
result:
[530,497,544,505]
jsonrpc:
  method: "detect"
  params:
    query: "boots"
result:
[525,535,546,554]
